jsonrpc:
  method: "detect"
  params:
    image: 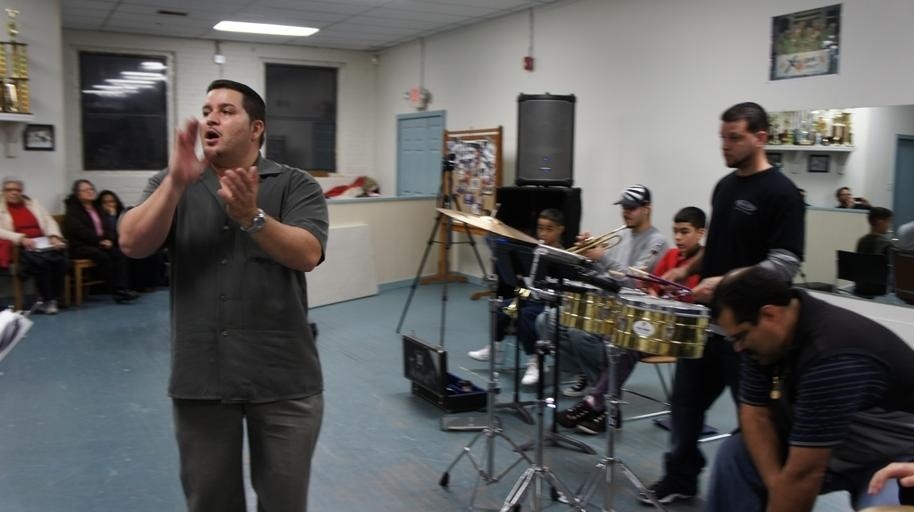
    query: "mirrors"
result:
[764,104,914,310]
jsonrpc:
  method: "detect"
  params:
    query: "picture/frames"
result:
[766,153,781,165]
[809,155,829,172]
[24,124,55,152]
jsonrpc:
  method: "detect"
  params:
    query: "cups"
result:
[832,123,845,141]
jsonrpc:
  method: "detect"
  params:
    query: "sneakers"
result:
[564,374,593,398]
[31,300,43,313]
[521,358,549,384]
[559,400,606,428]
[637,474,701,504]
[44,300,57,315]
[578,411,622,435]
[468,344,506,365]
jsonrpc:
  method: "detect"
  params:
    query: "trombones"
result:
[502,226,630,319]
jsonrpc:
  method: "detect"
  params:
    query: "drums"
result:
[612,294,713,360]
[532,244,596,299]
[559,278,646,336]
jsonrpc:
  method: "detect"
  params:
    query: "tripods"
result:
[480,237,538,426]
[436,274,535,511]
[395,152,495,347]
[574,341,667,512]
[513,253,597,455]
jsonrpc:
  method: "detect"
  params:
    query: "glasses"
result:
[4,188,22,192]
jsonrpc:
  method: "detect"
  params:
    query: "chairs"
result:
[7,216,71,306]
[613,352,678,423]
[52,215,104,305]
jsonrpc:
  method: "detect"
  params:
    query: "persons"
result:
[555,205,707,436]
[466,209,566,386]
[711,265,914,511]
[62,178,143,304]
[116,78,328,512]
[897,220,913,252]
[535,184,668,398]
[798,189,810,206]
[834,186,869,209]
[95,185,125,220]
[634,102,805,505]
[859,460,913,511]
[851,208,895,299]
[0,176,68,314]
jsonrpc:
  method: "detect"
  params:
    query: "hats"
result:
[614,185,651,207]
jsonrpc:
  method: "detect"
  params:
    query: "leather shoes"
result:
[114,291,140,303]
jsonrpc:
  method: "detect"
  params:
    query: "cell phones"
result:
[853,198,861,201]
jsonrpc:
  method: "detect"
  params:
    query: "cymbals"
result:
[436,208,543,244]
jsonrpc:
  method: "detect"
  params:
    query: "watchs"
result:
[239,208,266,233]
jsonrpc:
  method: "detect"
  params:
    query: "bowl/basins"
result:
[794,130,818,146]
